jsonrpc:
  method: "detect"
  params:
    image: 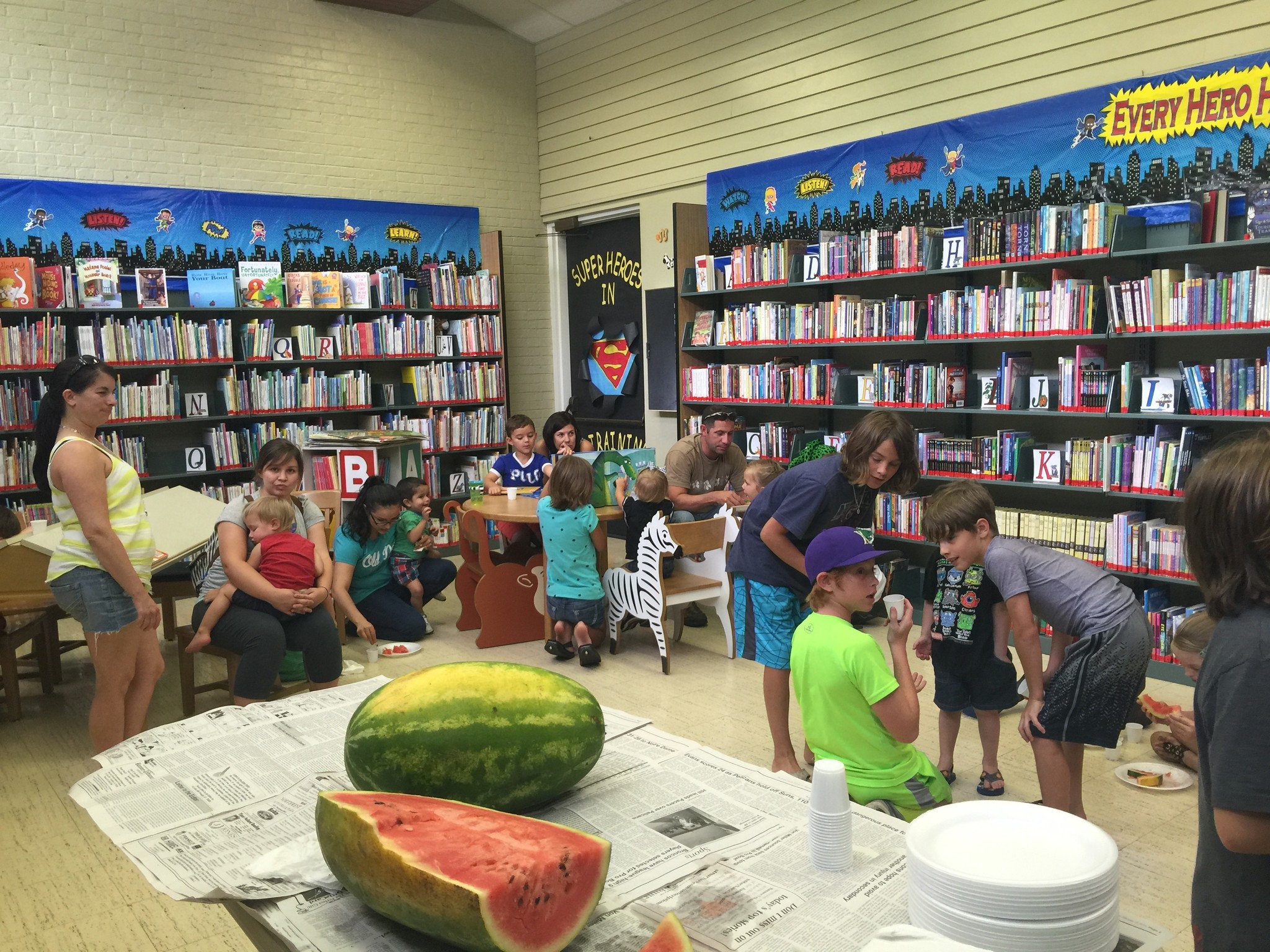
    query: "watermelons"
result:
[345,660,606,812]
[382,645,408,654]
[316,789,613,951]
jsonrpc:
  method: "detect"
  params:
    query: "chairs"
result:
[0,491,349,717]
[440,496,752,674]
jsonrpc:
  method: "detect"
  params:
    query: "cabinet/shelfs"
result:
[0,230,509,561]
[298,439,423,558]
[672,198,1270,689]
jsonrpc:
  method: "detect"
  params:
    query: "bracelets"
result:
[318,586,328,600]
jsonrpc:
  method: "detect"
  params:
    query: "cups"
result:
[1017,679,1045,699]
[883,594,905,620]
[366,648,379,663]
[507,487,517,500]
[1126,723,1143,742]
[809,759,853,871]
[468,480,485,506]
[30,519,47,535]
[1105,740,1123,761]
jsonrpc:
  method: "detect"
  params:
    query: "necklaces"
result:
[59,423,107,452]
[851,481,866,515]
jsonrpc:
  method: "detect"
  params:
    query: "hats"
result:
[805,525,903,583]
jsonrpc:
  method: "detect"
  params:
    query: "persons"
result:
[481,406,1216,824]
[1184,427,1270,951]
[33,355,457,769]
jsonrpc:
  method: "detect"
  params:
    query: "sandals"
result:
[936,764,957,785]
[977,768,1005,796]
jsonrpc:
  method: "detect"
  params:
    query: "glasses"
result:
[367,508,402,526]
[703,411,737,421]
[64,354,100,388]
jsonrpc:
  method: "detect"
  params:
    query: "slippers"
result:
[1150,730,1198,773]
[544,639,575,658]
[788,768,812,784]
[578,643,602,666]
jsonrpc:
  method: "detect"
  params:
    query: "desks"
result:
[464,488,625,644]
[213,680,1166,952]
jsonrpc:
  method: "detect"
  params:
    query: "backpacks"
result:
[184,494,304,598]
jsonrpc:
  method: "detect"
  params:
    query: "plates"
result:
[730,505,749,512]
[1115,762,1193,790]
[1084,736,1124,747]
[378,642,422,656]
[905,800,1120,952]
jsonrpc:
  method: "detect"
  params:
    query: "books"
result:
[681,189,1269,667]
[0,253,510,547]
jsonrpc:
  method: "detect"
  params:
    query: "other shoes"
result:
[683,606,708,628]
[620,614,650,632]
[433,591,446,601]
[422,614,434,635]
